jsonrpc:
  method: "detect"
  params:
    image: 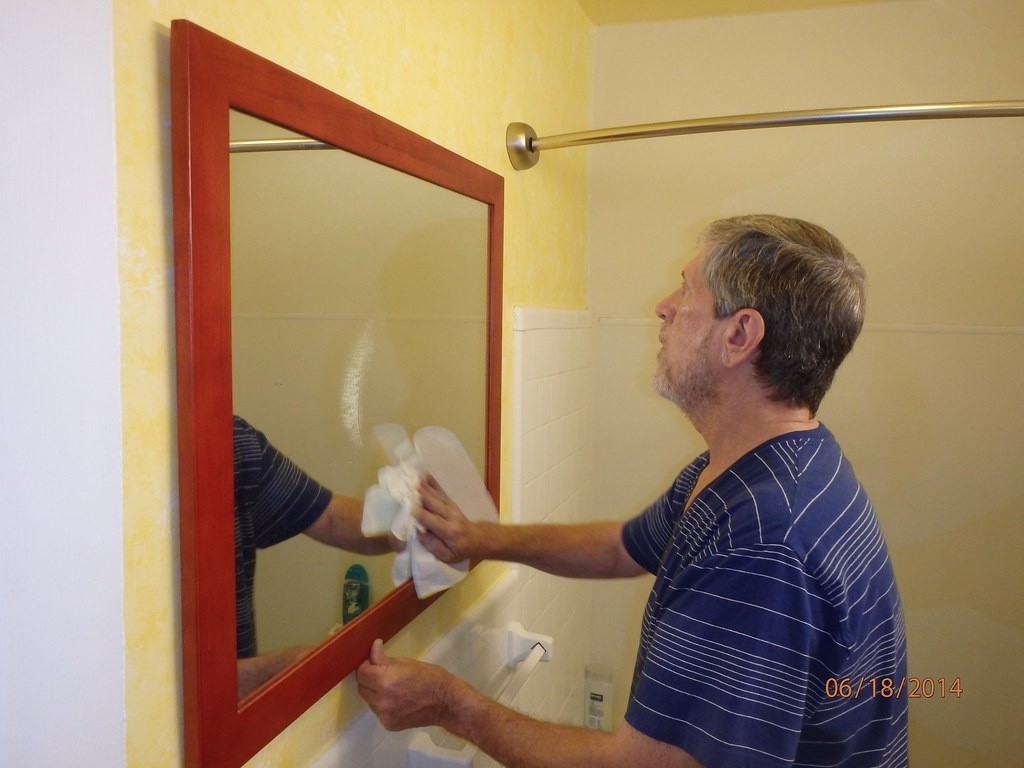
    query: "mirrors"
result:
[169,19,503,768]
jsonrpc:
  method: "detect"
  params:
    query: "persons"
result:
[356,212,911,768]
[231,413,406,706]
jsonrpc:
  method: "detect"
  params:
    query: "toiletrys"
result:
[583,663,615,735]
[340,563,368,627]
